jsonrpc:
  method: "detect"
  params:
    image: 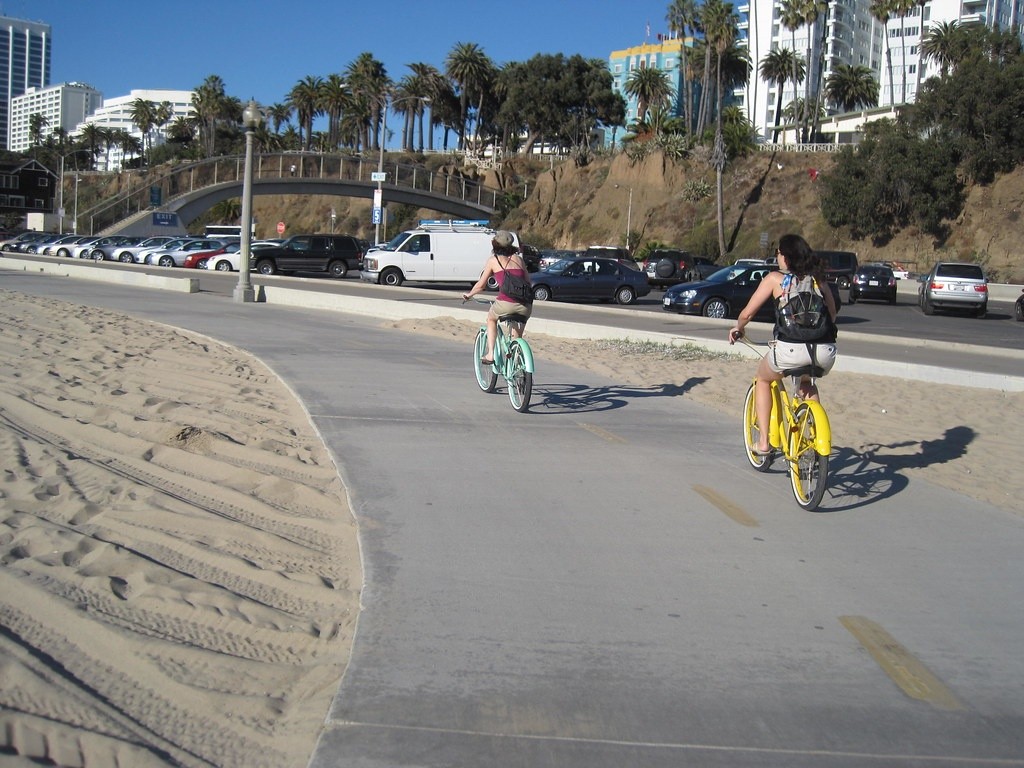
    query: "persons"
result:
[727,234,838,456]
[463,230,534,364]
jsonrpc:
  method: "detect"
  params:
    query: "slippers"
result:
[479,356,495,364]
[749,443,770,456]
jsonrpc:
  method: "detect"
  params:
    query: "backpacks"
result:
[779,268,829,341]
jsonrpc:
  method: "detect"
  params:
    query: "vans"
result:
[360,219,522,291]
[583,245,641,278]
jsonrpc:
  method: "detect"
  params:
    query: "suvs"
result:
[811,250,858,289]
[250,233,363,278]
[642,249,703,285]
[919,262,989,319]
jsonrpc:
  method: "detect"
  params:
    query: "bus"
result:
[205,225,256,240]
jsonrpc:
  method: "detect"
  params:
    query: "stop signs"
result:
[277,222,285,234]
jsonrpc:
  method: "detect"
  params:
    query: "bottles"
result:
[778,297,795,327]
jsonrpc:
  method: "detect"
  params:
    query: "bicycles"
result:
[730,330,832,511]
[462,296,534,413]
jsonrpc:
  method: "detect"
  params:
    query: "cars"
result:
[693,256,724,279]
[848,261,909,305]
[1015,289,1024,321]
[1,229,280,274]
[662,259,841,319]
[522,245,651,305]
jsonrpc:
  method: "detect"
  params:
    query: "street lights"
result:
[60,149,92,235]
[232,96,262,305]
[339,81,432,248]
[615,184,633,248]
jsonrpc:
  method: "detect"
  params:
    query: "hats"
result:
[494,231,514,247]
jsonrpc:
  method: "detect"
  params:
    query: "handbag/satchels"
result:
[494,253,533,304]
